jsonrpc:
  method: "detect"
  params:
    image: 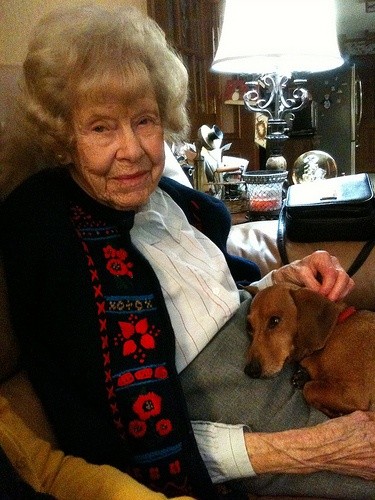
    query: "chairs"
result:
[0,63,375,500]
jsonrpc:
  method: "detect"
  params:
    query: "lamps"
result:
[208,0,347,200]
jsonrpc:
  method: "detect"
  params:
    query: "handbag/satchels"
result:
[274,169,374,290]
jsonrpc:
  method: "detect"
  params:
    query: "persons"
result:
[0,4,375,500]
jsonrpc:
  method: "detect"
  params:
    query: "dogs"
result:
[243,282,375,418]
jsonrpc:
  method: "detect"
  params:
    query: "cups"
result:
[241,169,289,222]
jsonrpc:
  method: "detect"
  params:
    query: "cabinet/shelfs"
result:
[147,0,260,171]
